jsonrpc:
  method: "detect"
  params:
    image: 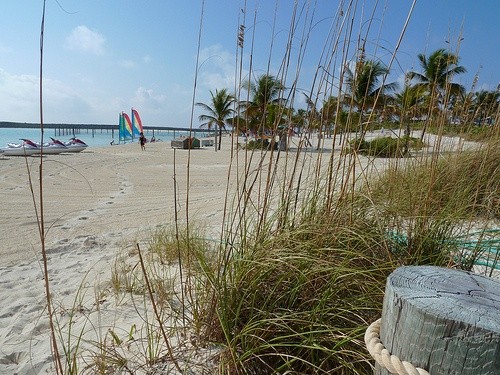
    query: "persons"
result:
[138,132,146,151]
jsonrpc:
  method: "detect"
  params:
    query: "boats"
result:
[33,136,67,154]
[2,139,38,155]
[61,137,88,153]
